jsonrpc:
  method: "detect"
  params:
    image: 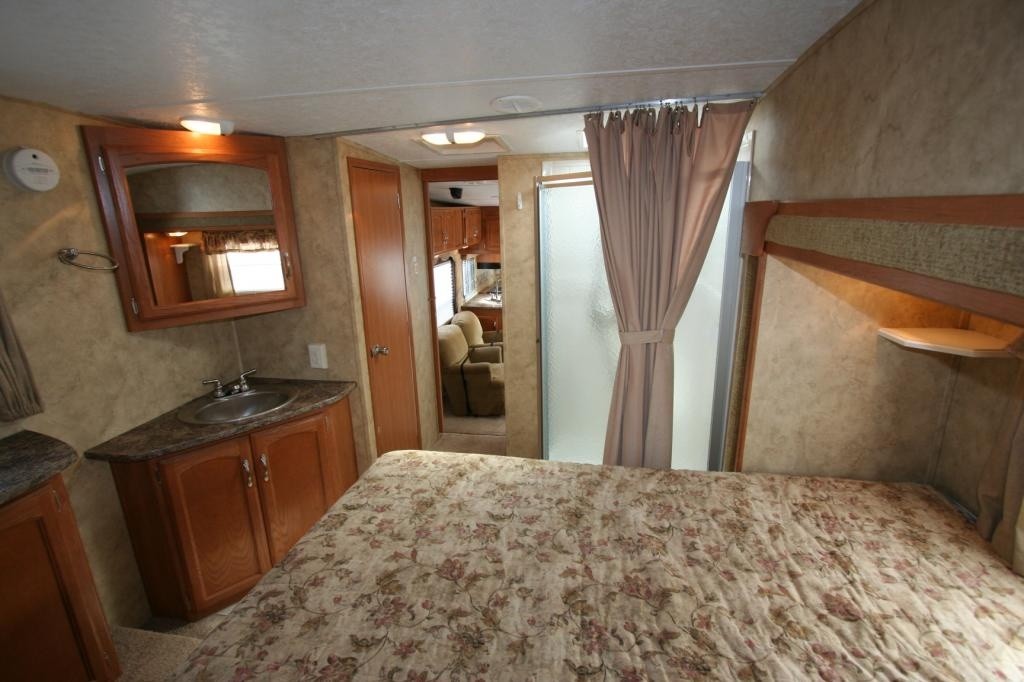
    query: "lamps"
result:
[179,115,234,136]
[420,127,486,145]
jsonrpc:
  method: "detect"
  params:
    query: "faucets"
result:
[229,384,241,395]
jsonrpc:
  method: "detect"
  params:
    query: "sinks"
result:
[195,391,289,421]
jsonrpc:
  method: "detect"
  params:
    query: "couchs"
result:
[438,311,505,417]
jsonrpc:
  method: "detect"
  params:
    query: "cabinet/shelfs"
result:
[430,206,500,259]
[109,398,358,623]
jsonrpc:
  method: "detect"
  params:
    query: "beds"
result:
[164,195,1024,682]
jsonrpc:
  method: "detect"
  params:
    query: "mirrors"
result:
[103,144,298,318]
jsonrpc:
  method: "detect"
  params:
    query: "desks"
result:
[0,430,125,682]
